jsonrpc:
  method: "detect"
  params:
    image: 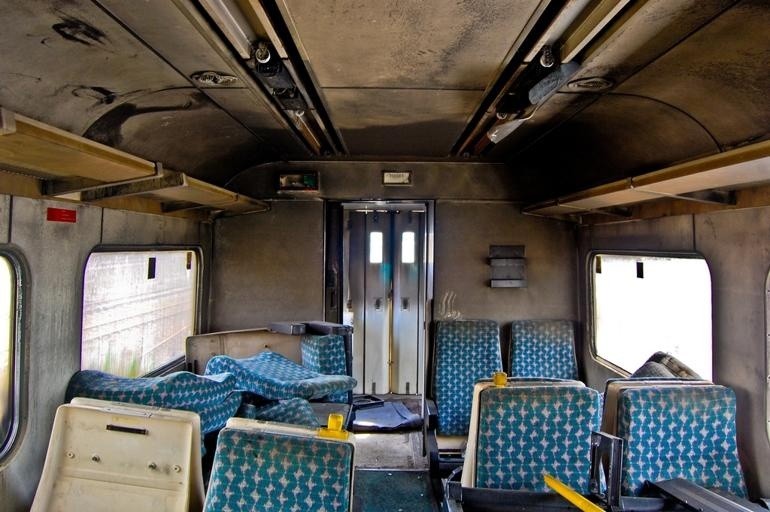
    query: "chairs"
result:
[603,377,748,510]
[423,321,505,497]
[28,397,357,511]
[440,373,606,511]
[508,319,580,384]
